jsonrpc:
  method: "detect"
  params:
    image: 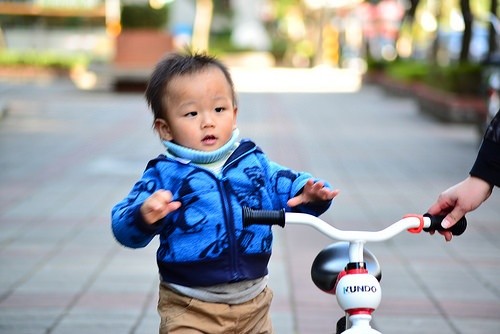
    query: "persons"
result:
[111,54,341,334]
[428,109,500,242]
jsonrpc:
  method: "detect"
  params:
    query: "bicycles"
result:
[240,202,467,334]
